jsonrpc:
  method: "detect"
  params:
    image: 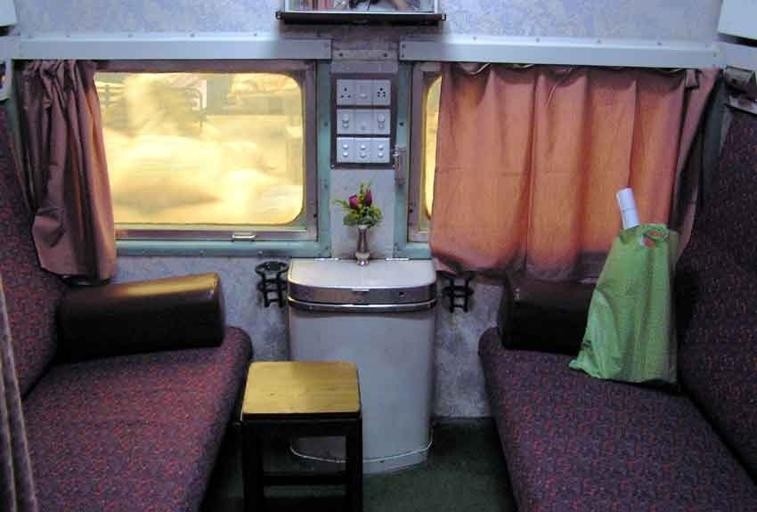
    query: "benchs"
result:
[477,93,757,512]
[0,104,254,512]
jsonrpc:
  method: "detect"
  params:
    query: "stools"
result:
[241,359,364,512]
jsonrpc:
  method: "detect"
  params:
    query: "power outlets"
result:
[336,79,392,107]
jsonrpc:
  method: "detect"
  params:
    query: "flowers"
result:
[331,182,384,226]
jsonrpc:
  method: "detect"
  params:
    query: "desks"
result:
[287,258,437,475]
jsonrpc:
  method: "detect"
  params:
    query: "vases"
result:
[355,223,370,265]
